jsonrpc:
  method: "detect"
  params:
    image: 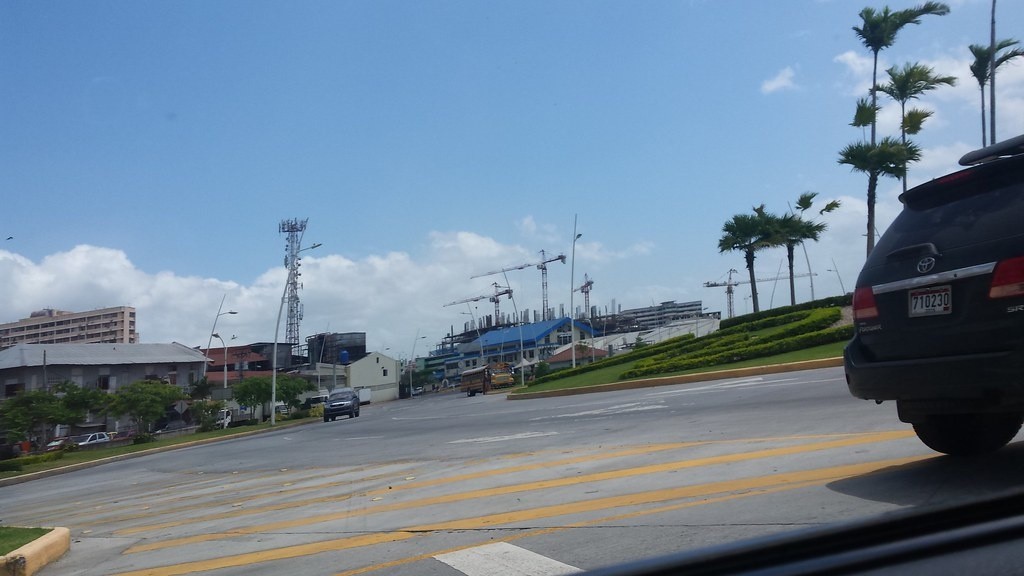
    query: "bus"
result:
[459,360,515,397]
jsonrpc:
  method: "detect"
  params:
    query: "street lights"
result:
[203,294,239,377]
[410,328,426,399]
[269,242,325,427]
[570,214,583,369]
[211,332,239,428]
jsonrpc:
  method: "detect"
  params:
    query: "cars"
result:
[842,132,1024,454]
[324,391,361,423]
[42,393,328,453]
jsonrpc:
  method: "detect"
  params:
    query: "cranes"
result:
[702,267,819,319]
[573,271,594,321]
[470,249,567,321]
[442,282,514,326]
